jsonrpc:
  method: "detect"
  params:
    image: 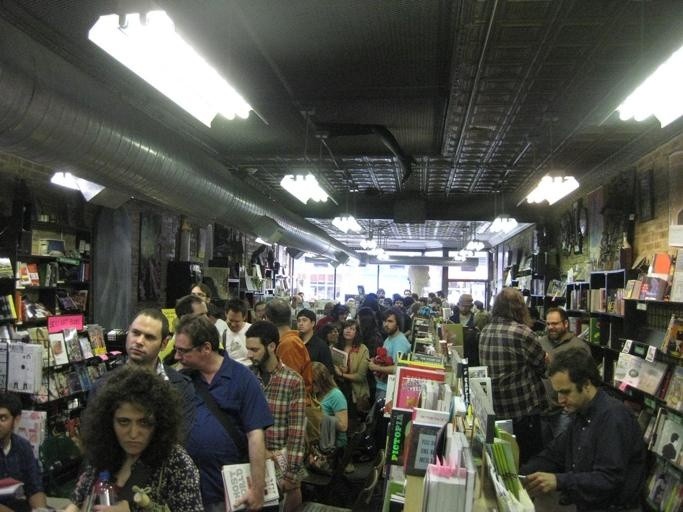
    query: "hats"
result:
[458,293,473,307]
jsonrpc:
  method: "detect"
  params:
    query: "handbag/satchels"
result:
[304,442,338,476]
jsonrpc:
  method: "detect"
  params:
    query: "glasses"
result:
[172,345,200,353]
[189,293,208,298]
[544,320,563,327]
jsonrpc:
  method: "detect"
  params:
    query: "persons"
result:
[1,284,649,510]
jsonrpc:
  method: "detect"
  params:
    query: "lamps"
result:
[596,45,683,130]
[332,167,362,235]
[278,105,339,208]
[487,170,520,233]
[513,109,581,206]
[79,0,270,132]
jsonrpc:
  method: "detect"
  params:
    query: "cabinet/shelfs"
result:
[1,177,96,334]
[0,323,128,512]
[493,243,545,319]
[558,248,683,512]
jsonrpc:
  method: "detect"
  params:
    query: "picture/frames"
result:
[637,169,655,224]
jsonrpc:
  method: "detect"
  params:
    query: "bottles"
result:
[86,471,119,512]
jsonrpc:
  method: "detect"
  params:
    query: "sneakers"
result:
[345,461,357,475]
